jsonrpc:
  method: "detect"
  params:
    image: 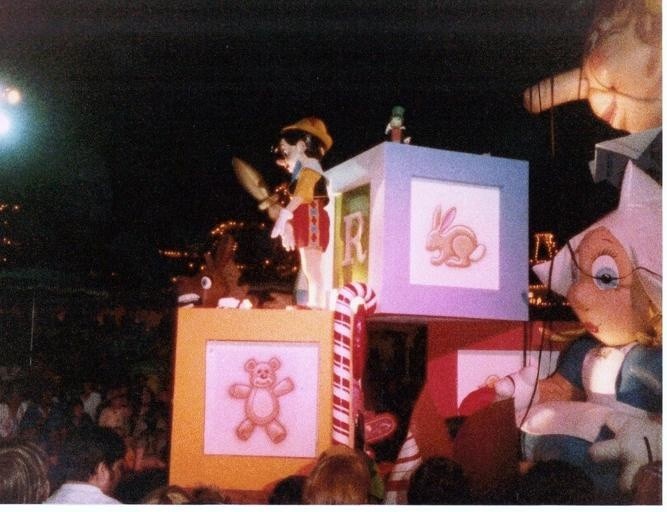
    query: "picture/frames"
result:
[166,304,336,492]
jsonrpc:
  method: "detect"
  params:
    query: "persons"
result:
[265,115,334,306]
[383,106,416,146]
[0,274,661,503]
[491,157,662,492]
